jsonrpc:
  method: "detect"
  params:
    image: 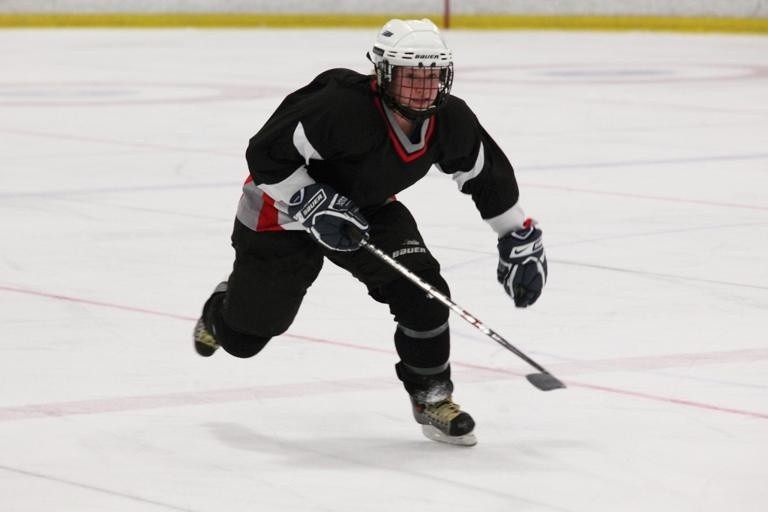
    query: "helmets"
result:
[366,18,454,89]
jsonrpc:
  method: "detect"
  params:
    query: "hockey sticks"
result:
[346,228,567,391]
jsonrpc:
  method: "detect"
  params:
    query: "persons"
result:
[193,19,547,436]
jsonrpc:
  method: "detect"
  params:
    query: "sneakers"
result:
[413,393,475,437]
[194,281,228,357]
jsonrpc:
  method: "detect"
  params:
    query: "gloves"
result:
[288,183,370,252]
[497,218,548,308]
